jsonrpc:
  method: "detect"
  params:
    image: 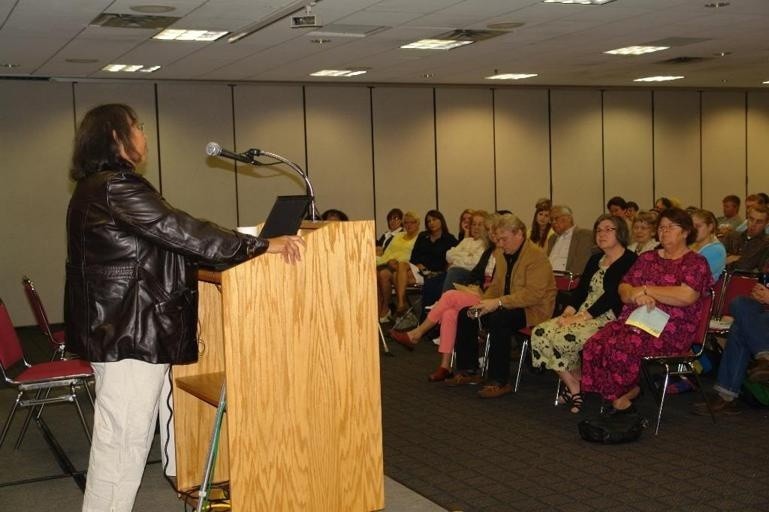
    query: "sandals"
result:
[557,386,572,406]
[570,392,586,415]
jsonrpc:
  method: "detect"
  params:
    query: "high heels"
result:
[380,309,392,323]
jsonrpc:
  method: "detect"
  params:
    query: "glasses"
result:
[658,223,683,231]
[594,227,617,234]
[548,215,569,223]
[388,216,399,222]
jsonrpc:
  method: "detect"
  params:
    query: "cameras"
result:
[470,309,479,319]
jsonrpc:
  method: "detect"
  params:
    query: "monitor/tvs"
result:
[259,195,314,239]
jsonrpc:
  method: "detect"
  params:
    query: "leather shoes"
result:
[630,389,645,404]
[601,403,638,414]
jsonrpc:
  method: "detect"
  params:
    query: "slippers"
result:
[388,328,416,352]
[427,366,453,383]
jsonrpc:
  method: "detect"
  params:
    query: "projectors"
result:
[288,14,324,29]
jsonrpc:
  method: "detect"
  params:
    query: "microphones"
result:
[206,142,262,167]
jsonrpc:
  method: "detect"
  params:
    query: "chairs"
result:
[450,320,489,375]
[643,289,720,438]
[716,267,769,383]
[0,299,96,455]
[388,282,431,335]
[712,272,728,316]
[516,278,580,407]
[480,269,572,392]
[21,273,99,420]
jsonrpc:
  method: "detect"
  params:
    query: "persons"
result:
[62,100,306,512]
[314,190,769,428]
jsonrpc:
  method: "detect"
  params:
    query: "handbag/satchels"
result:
[578,415,649,444]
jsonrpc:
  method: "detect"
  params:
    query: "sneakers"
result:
[477,382,515,399]
[688,392,746,418]
[443,372,482,387]
[745,356,769,386]
